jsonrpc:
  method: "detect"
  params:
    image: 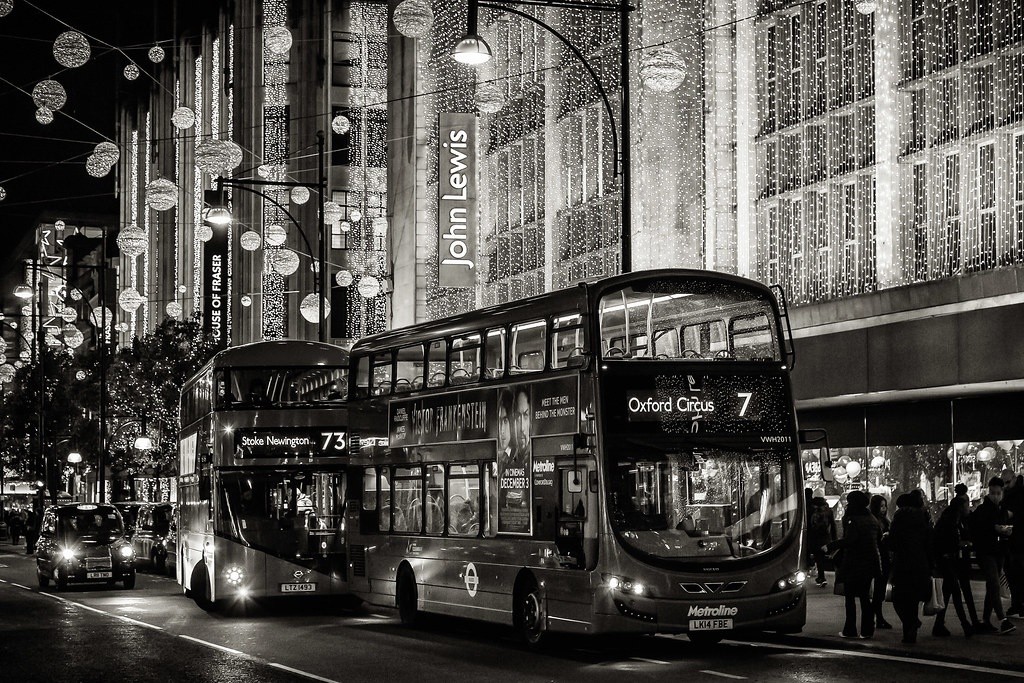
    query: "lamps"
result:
[0,0,878,381]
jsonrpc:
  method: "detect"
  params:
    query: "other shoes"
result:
[901,638,916,644]
[876,620,892,629]
[983,622,998,632]
[1006,606,1019,616]
[838,632,858,638]
[972,624,990,635]
[859,635,873,639]
[816,578,820,586]
[1001,624,1017,633]
[932,625,951,636]
[819,578,827,587]
[965,627,980,637]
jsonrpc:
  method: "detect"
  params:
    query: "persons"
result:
[497,387,530,508]
[288,488,315,527]
[380,485,479,534]
[805,469,1024,644]
[0,508,43,555]
[676,476,736,558]
[233,484,264,516]
[744,472,786,550]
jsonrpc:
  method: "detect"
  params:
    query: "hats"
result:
[950,484,970,511]
[896,491,921,509]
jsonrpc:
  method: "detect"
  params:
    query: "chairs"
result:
[382,494,489,537]
[309,347,733,400]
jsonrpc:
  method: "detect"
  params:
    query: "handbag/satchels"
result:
[923,576,946,616]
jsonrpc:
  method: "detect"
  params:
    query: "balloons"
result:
[800,449,885,484]
[948,440,1024,467]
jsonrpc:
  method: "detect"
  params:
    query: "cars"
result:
[111,499,177,578]
[31,502,136,593]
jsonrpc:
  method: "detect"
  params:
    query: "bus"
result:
[175,338,351,614]
[345,262,837,654]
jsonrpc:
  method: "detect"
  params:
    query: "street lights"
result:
[203,128,329,344]
[446,0,638,273]
[11,224,110,505]
